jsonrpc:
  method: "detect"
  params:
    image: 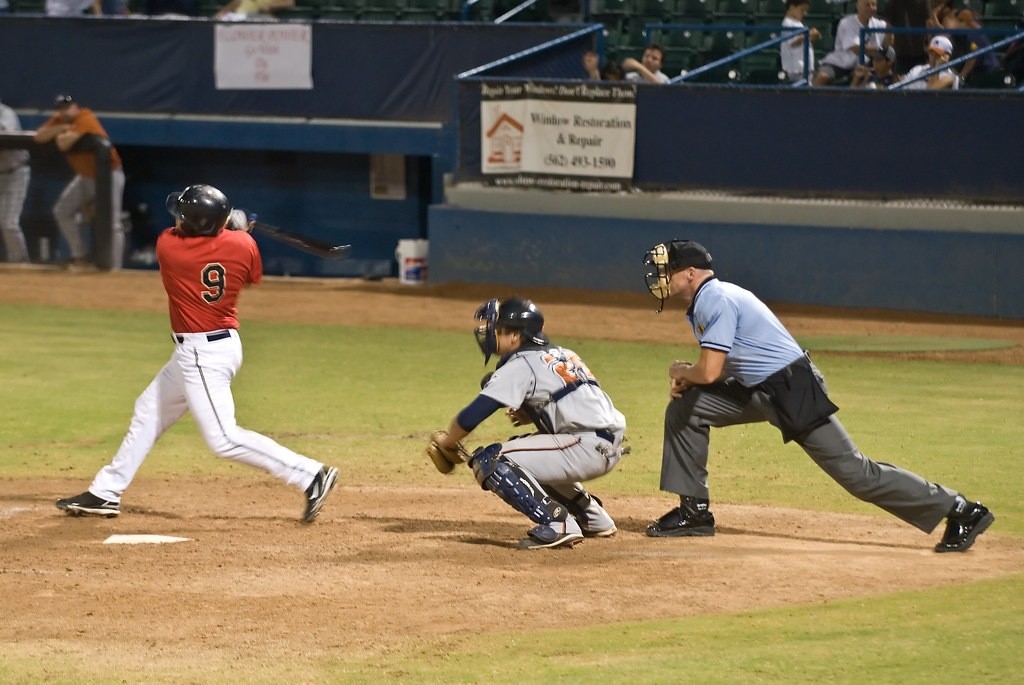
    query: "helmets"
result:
[499,297,550,345]
[165,185,231,237]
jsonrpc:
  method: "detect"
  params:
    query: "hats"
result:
[54,94,72,108]
[665,238,713,269]
[928,35,953,57]
[880,45,896,63]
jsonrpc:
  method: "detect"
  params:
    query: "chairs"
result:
[4,0,1024,80]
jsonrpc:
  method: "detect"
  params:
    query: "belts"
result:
[803,349,811,362]
[595,429,614,444]
[170,331,231,343]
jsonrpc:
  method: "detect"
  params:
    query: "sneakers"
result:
[305,465,339,522]
[56,492,121,518]
[516,530,584,551]
[575,518,617,538]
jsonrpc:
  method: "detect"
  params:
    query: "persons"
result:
[622,44,671,85]
[811,0,894,88]
[849,44,901,89]
[431,295,625,550]
[56,184,340,524]
[925,0,1002,86]
[781,0,821,87]
[887,35,960,91]
[646,239,995,552]
[584,51,626,81]
[32,93,127,273]
[0,103,31,263]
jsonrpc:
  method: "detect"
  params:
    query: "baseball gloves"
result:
[424,430,471,475]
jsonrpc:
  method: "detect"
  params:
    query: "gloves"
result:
[226,209,249,232]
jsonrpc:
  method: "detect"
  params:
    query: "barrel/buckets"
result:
[396,238,429,286]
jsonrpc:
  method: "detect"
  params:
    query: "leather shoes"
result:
[646,495,715,537]
[935,494,994,553]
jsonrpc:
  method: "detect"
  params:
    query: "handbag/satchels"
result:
[762,355,839,444]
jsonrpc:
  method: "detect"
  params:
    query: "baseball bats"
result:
[247,220,341,258]
